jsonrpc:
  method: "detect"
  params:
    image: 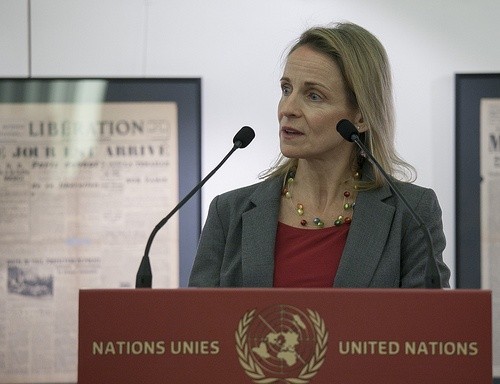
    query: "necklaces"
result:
[285,168,361,227]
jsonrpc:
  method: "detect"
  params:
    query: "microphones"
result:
[336,118,441,288]
[135,125,255,289]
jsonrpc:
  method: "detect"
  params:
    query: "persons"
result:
[188,22,450,289]
